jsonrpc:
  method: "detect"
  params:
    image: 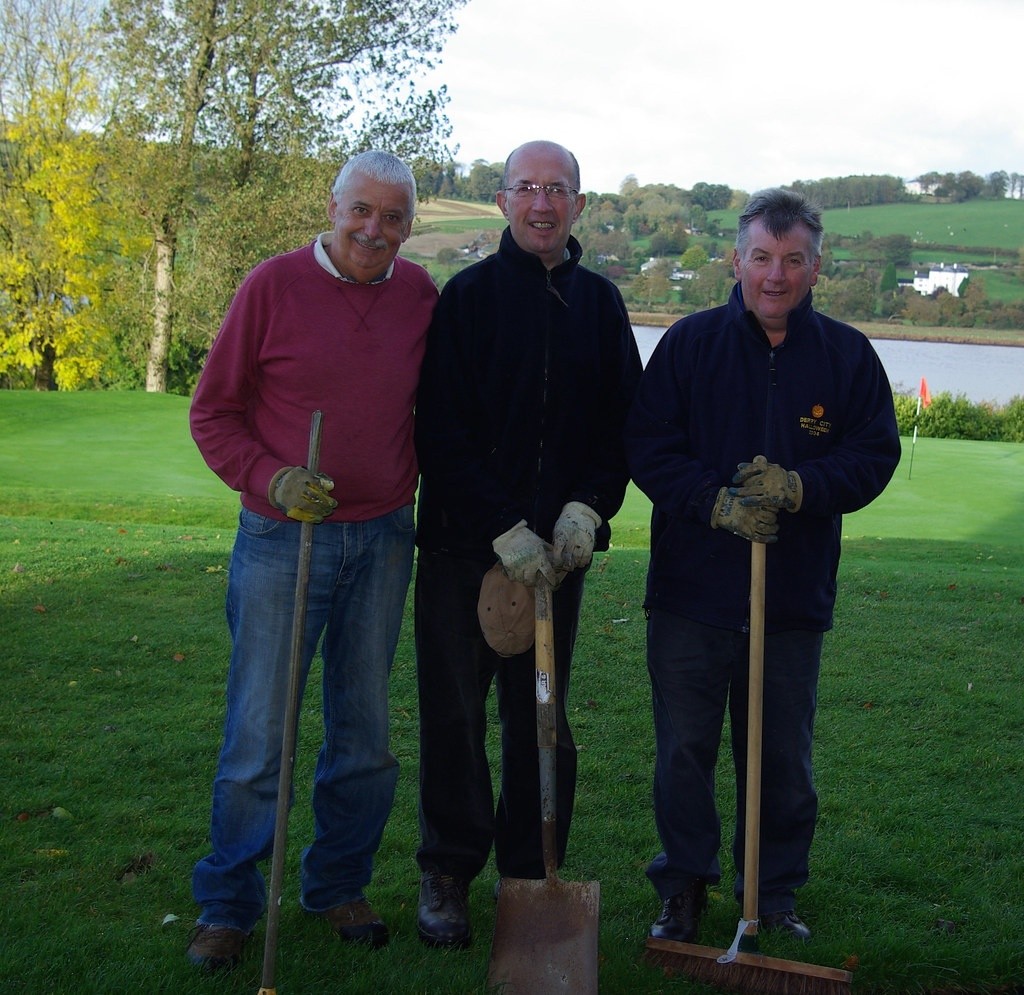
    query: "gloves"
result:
[728,459,803,515]
[708,485,780,545]
[267,465,339,524]
[550,501,603,573]
[491,519,560,599]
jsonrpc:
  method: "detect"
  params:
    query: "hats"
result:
[477,538,572,660]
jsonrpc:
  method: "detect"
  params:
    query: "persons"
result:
[622,188,902,941]
[413,140,644,950]
[184,151,442,964]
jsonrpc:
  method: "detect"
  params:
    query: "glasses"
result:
[504,182,580,200]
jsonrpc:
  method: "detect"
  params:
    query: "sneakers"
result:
[186,921,252,973]
[763,907,813,942]
[328,898,392,948]
[414,870,475,952]
[649,894,711,946]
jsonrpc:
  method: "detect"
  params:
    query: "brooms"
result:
[638,455,854,994]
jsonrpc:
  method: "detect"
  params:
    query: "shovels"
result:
[485,567,601,995]
